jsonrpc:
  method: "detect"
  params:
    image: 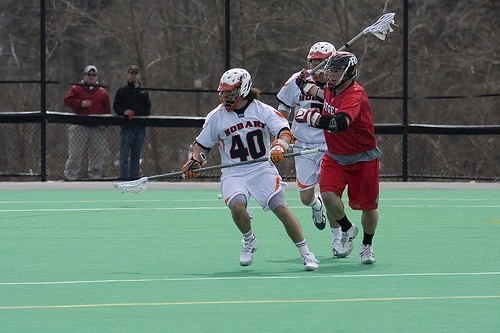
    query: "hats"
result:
[128,65,140,74]
[84,65,98,75]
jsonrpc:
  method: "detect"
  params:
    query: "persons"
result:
[276,42,342,256]
[112,65,152,181]
[181,67,320,272]
[295,52,382,265]
[63,65,111,180]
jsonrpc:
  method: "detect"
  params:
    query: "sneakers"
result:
[239,232,258,266]
[311,196,326,230]
[331,238,341,256]
[337,223,359,258]
[358,241,376,263]
[301,252,319,271]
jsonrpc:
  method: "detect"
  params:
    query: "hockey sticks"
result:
[111,147,319,197]
[309,12,399,76]
[288,143,328,153]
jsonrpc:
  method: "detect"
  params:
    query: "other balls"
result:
[217,194,223,199]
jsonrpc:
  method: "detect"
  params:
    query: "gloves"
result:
[295,108,319,128]
[268,139,289,166]
[295,68,316,95]
[181,159,201,179]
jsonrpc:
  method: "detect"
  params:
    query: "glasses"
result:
[87,74,97,76]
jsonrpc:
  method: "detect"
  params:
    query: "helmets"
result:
[323,52,359,96]
[307,41,336,82]
[216,67,252,112]
